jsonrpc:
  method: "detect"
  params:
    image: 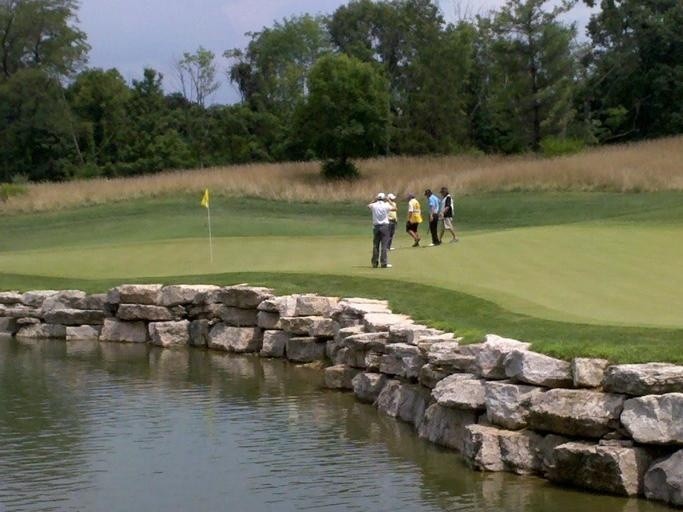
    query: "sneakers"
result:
[385,264,391,268]
[388,238,459,251]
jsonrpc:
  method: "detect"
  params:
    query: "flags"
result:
[199,189,208,210]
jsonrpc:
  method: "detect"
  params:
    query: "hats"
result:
[424,189,431,196]
[440,188,448,194]
[376,193,384,200]
[387,193,396,200]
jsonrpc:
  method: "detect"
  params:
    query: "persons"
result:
[404,192,423,247]
[383,193,398,252]
[424,187,443,248]
[438,186,459,243]
[366,192,400,268]
